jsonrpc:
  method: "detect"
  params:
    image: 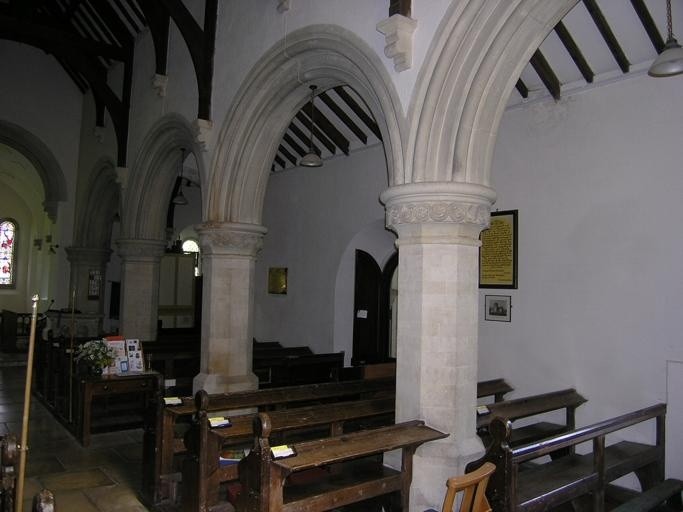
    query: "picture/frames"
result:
[477,208,519,323]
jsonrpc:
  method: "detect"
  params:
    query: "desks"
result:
[31,337,163,448]
[476,378,683,512]
[141,337,397,399]
[139,391,451,512]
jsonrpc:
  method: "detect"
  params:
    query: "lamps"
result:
[171,148,189,206]
[298,84,325,168]
[646,2,682,79]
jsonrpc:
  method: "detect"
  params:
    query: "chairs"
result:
[441,461,495,512]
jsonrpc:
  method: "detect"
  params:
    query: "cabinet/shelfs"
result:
[156,253,196,329]
